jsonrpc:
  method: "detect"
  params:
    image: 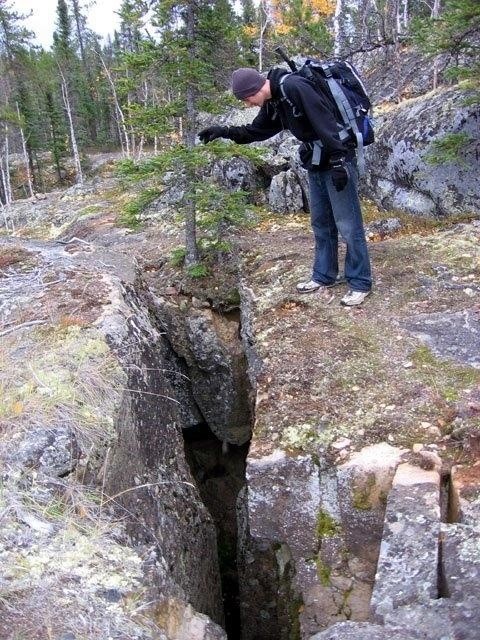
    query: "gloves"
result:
[198,125,228,144]
[295,60,375,146]
[331,161,347,192]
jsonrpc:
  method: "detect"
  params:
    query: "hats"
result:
[231,67,266,97]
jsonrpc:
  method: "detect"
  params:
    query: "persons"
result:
[197,68,375,306]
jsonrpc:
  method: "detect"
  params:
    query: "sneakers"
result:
[296,278,336,293]
[340,289,371,306]
